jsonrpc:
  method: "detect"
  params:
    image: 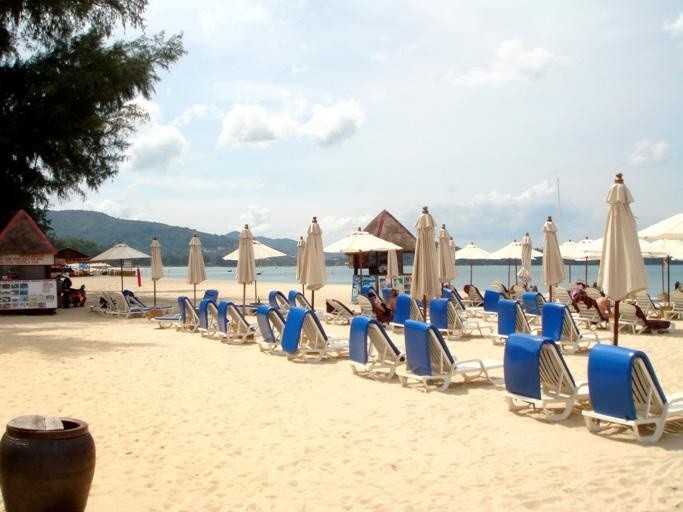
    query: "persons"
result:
[596,290,611,317]
[366,290,400,327]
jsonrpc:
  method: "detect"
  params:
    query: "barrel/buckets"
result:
[0,418,95,511]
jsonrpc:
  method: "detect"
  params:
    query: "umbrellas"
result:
[186,232,207,308]
[595,174,650,349]
[237,225,255,320]
[517,231,531,289]
[451,241,489,289]
[541,216,564,304]
[433,219,456,303]
[293,235,305,300]
[148,235,164,308]
[221,239,287,303]
[406,203,439,323]
[322,226,404,295]
[481,210,683,306]
[87,240,151,293]
[303,216,324,314]
[447,232,456,263]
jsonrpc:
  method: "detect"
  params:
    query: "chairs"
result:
[155,289,363,363]
[503,332,591,423]
[90,287,173,320]
[581,343,683,443]
[396,319,504,392]
[355,281,683,354]
[350,316,459,382]
